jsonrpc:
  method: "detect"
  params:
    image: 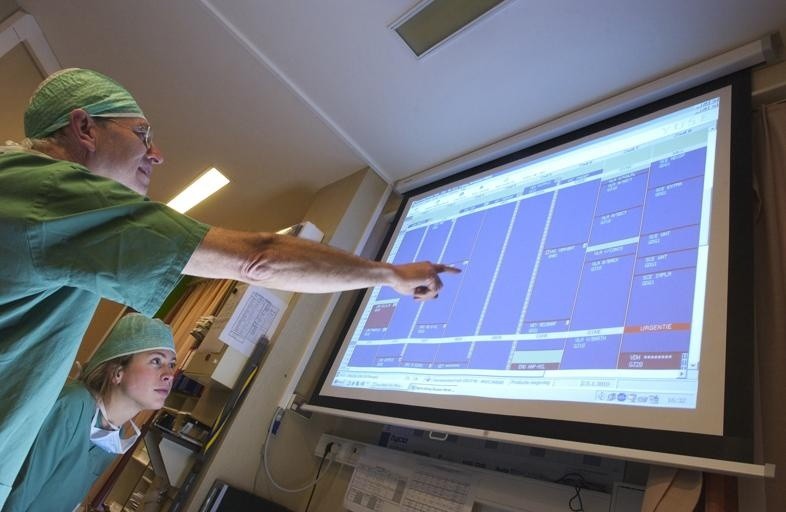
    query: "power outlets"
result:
[314,434,349,464]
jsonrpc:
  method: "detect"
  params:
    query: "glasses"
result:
[106,118,154,149]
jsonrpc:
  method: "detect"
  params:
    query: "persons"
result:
[0,66,463,512]
[3,311,179,512]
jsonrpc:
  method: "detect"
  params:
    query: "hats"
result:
[24,64,145,138]
[78,313,178,381]
